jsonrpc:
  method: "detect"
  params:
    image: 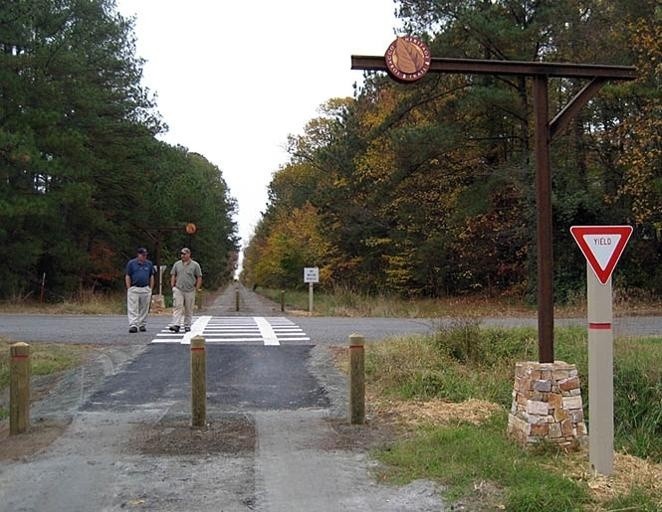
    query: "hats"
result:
[137,247,149,255]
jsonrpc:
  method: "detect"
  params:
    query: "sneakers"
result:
[140,326,146,331]
[129,327,137,332]
[185,327,191,331]
[169,325,181,332]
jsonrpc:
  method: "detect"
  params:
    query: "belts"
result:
[132,283,149,287]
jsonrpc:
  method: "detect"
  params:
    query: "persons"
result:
[124,247,155,334]
[167,247,204,334]
